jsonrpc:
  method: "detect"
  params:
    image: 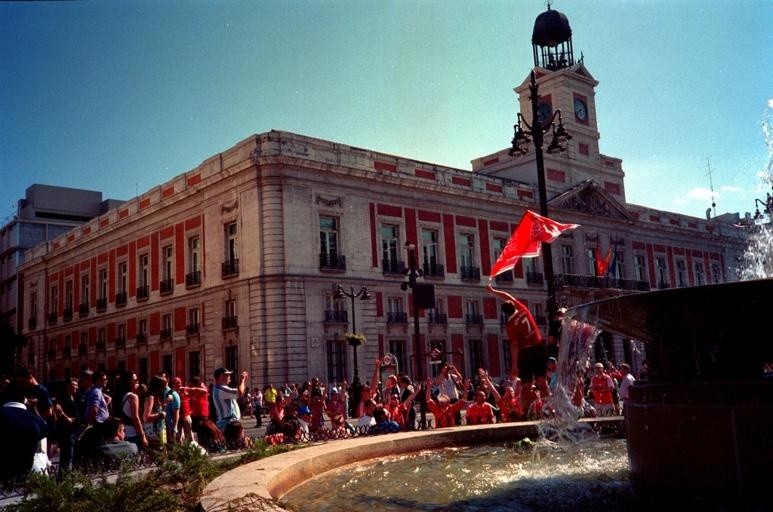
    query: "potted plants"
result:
[345,334,366,346]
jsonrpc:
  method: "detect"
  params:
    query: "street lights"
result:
[505,68,572,348]
[401,242,436,429]
[332,281,363,414]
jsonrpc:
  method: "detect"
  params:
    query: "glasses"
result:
[133,379,137,384]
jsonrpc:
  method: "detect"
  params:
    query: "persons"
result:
[486,283,557,421]
[243,359,635,444]
[0,359,248,484]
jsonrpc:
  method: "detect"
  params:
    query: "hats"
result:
[596,362,602,367]
[214,367,233,374]
[547,357,556,362]
[619,364,630,370]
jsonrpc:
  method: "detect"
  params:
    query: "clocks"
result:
[574,98,588,121]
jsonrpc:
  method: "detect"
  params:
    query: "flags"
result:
[594,244,619,278]
[487,208,582,283]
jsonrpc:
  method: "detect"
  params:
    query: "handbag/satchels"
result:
[298,406,311,414]
